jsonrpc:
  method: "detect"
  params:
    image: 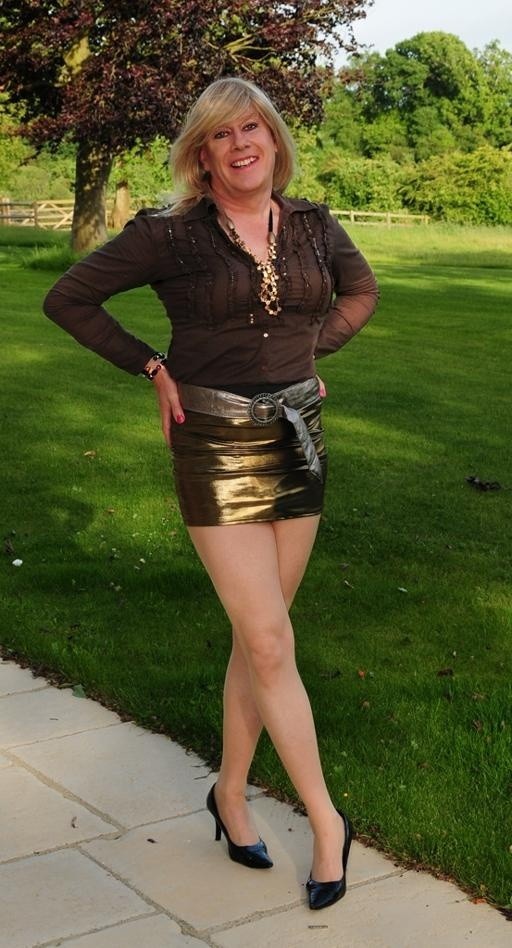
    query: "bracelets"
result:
[142,351,168,381]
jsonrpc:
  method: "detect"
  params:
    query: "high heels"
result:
[206,783,275,869]
[305,809,352,909]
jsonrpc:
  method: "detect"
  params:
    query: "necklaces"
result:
[223,206,286,318]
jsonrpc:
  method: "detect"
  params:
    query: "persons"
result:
[37,76,381,913]
